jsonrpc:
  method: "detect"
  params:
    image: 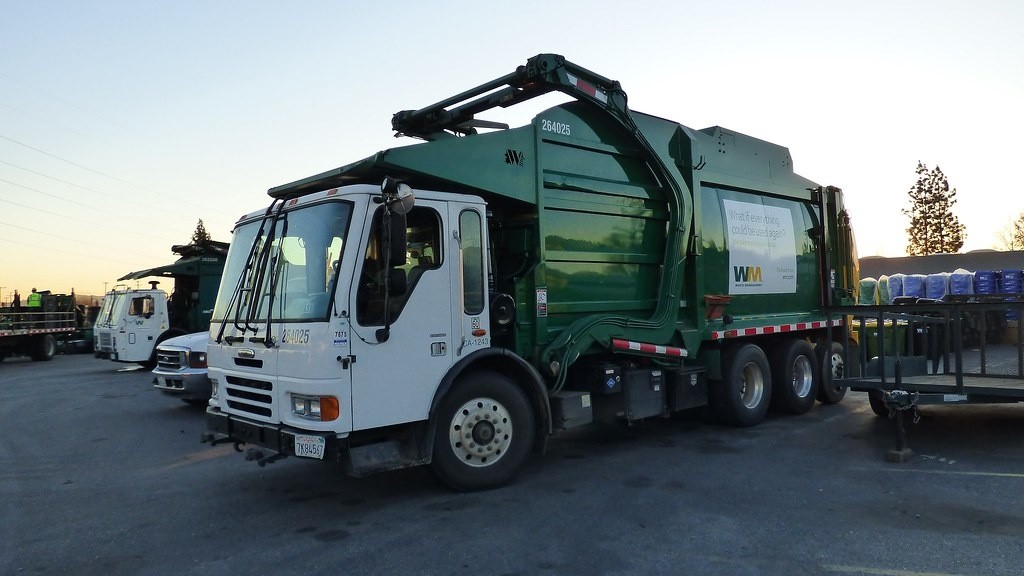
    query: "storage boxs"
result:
[860,268,1021,320]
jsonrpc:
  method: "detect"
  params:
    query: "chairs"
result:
[407,256,443,294]
[367,268,414,326]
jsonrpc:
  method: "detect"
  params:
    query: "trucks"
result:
[92,239,231,369]
[1,304,79,362]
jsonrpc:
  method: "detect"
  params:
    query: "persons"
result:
[27,288,46,327]
[9,289,21,329]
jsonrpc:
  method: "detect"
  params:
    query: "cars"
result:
[150,330,214,405]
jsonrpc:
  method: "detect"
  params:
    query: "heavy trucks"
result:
[204,52,862,486]
